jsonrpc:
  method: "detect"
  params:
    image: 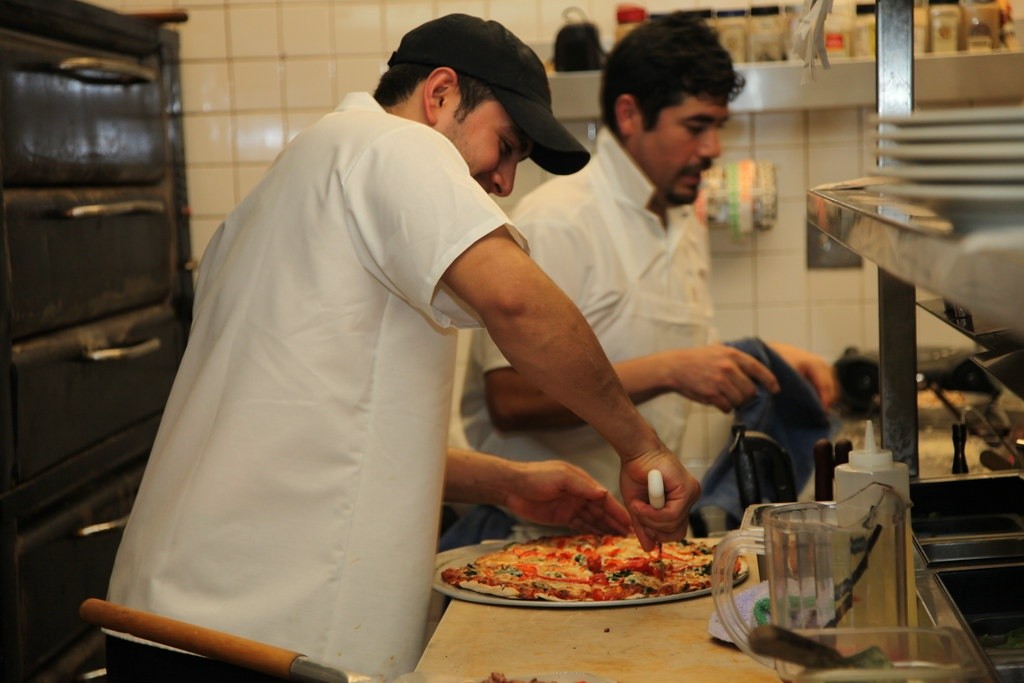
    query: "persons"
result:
[101,11,703,683]
[459,12,840,542]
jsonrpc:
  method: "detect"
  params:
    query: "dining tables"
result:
[412,538,960,683]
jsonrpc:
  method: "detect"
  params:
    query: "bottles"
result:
[617,7,646,44]
[833,420,919,659]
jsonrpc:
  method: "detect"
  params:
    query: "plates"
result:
[867,107,1024,233]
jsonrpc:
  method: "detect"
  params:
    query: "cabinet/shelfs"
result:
[806,0,1024,683]
[0,0,194,683]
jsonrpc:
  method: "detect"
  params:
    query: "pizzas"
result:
[439,530,742,602]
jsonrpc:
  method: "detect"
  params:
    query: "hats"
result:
[388,12,593,176]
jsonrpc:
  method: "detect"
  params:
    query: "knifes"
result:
[647,470,665,555]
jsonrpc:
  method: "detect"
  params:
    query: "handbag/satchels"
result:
[550,9,610,72]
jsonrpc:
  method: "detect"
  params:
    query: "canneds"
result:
[614,0,1004,65]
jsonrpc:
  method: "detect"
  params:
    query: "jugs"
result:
[711,482,909,683]
